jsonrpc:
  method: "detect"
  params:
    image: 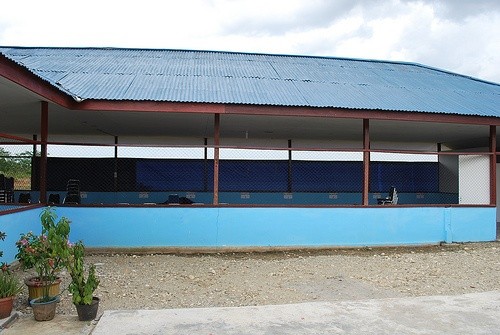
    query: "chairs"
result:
[377,184,400,207]
[1,174,85,207]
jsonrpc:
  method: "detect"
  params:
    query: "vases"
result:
[24,274,63,303]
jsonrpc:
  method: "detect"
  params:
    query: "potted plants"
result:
[30,246,64,321]
[62,240,101,322]
[0,271,26,318]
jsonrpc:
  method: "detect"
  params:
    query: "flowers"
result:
[15,204,73,277]
